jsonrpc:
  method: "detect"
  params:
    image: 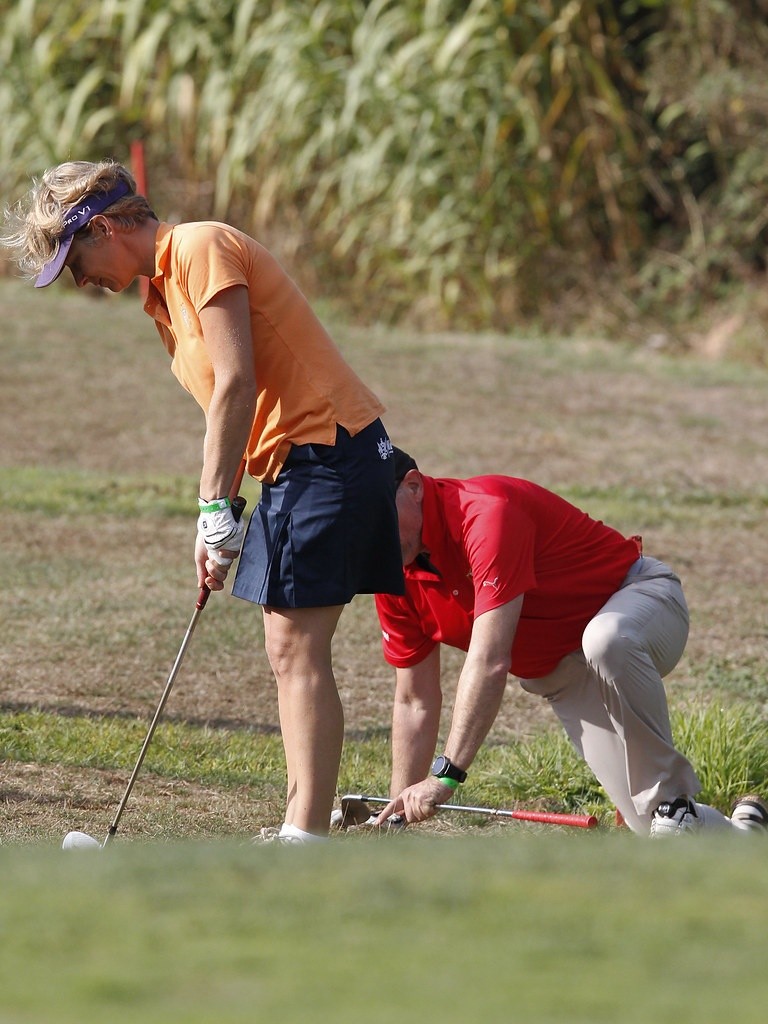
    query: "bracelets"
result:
[198,498,231,512]
[428,773,459,790]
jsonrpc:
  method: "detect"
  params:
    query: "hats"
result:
[34,174,130,288]
[392,446,418,490]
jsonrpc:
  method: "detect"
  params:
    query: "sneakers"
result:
[731,795,768,834]
[648,793,703,840]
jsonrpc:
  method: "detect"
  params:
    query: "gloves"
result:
[329,810,404,832]
[197,497,244,565]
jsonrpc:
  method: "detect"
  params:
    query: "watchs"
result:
[432,755,467,783]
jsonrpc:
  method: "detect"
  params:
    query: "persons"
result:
[3,158,409,842]
[375,446,768,838]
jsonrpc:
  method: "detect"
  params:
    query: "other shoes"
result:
[238,827,302,846]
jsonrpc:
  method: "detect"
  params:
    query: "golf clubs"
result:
[340,794,599,829]
[62,496,249,855]
